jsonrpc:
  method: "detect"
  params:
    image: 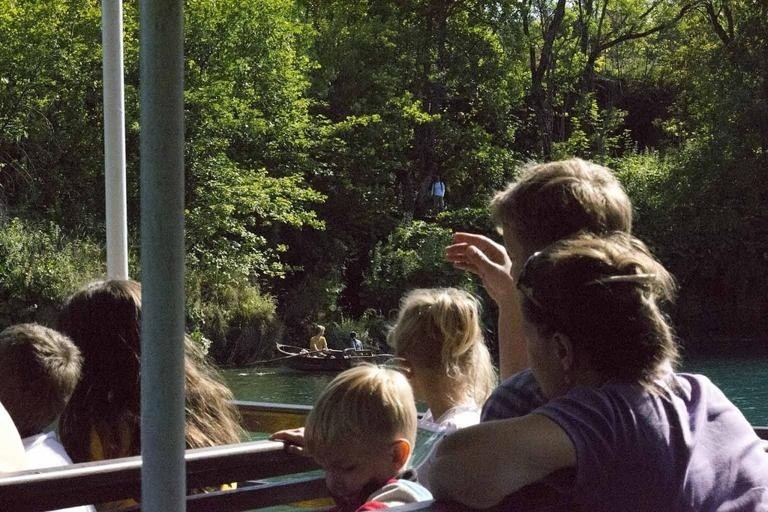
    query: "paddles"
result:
[244,349,326,367]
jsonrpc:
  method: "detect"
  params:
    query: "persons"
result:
[385,286,499,494]
[0,322,98,512]
[428,227,768,512]
[310,325,329,356]
[444,158,632,380]
[430,174,445,211]
[57,278,254,512]
[349,332,364,355]
[269,357,434,512]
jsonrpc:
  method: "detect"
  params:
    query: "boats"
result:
[275,340,394,370]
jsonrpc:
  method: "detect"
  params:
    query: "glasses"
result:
[513,245,560,321]
